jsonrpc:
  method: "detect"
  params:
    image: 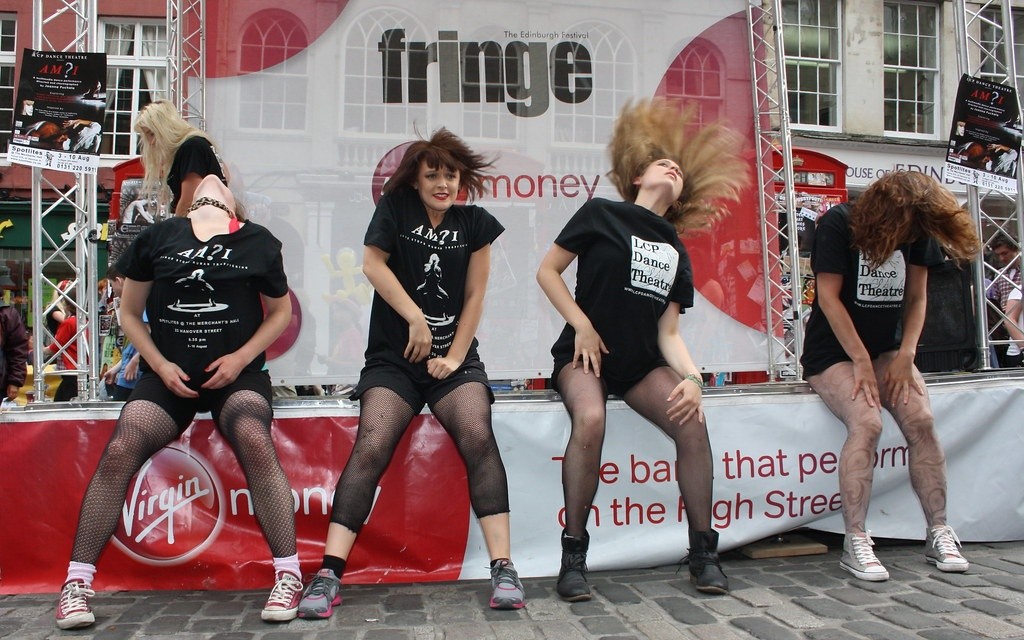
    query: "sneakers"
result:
[484,560,525,608]
[839,532,889,580]
[261,570,304,620]
[298,569,342,618]
[56,578,95,629]
[924,525,968,571]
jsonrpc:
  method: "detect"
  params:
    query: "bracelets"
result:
[686,374,703,389]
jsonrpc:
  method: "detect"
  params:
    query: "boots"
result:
[675,528,728,594]
[557,526,592,601]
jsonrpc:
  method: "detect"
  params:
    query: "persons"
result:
[29,276,89,402]
[294,127,525,621]
[0,289,28,406]
[136,101,227,217]
[98,261,151,400]
[536,94,753,604]
[799,169,977,583]
[978,229,1024,369]
[53,172,307,631]
